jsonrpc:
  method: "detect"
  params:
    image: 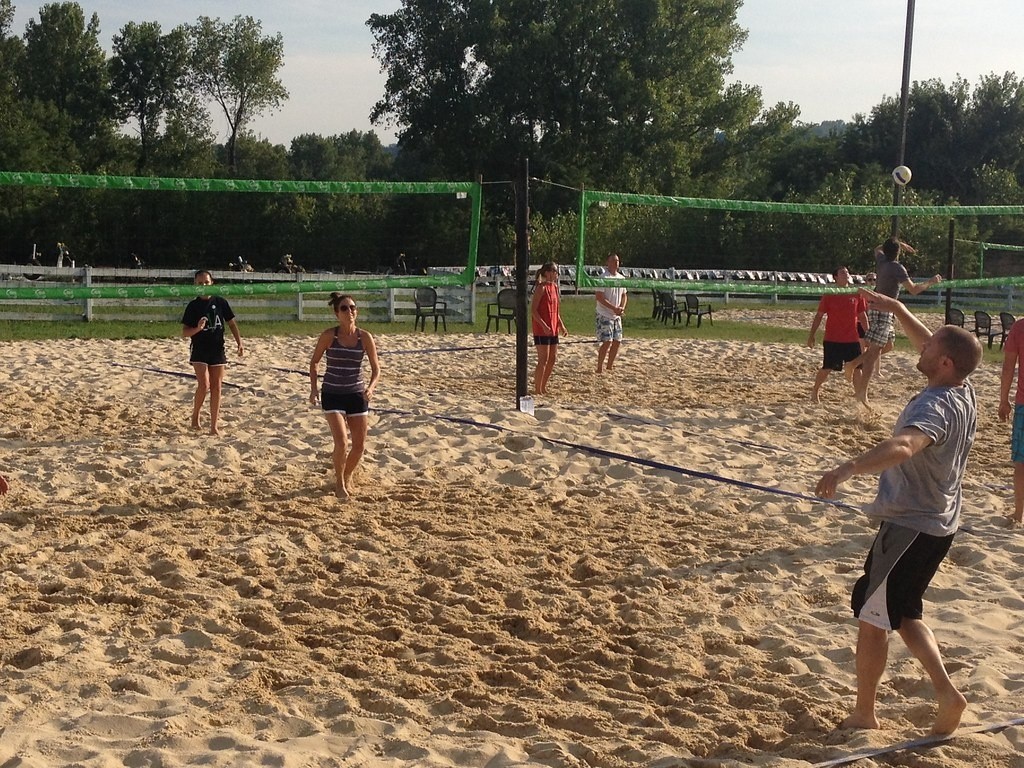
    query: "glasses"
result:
[339,305,357,311]
[866,278,877,282]
[546,268,560,276]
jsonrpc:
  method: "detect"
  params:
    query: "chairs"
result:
[949,308,974,334]
[414,286,448,334]
[974,311,1002,349]
[998,313,1016,350]
[651,288,714,328]
[486,289,517,335]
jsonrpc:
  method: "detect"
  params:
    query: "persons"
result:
[310,295,380,497]
[808,238,942,410]
[181,270,244,436]
[998,317,1024,522]
[816,288,983,734]
[531,262,568,394]
[595,254,627,373]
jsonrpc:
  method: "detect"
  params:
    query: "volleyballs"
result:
[892,166,912,186]
